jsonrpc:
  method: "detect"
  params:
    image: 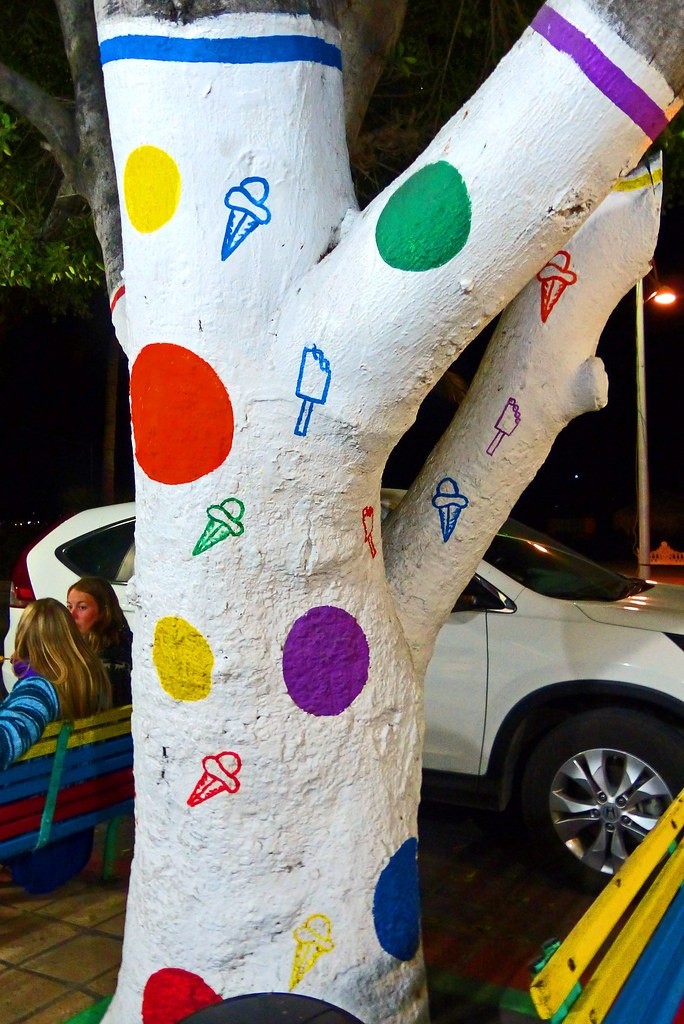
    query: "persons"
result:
[0,598,110,767]
[65,577,131,707]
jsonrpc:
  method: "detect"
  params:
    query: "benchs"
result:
[0,704,136,887]
[528,788,684,1024]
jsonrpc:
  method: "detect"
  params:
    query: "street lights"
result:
[635,287,678,581]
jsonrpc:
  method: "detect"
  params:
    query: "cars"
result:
[0,488,684,892]
